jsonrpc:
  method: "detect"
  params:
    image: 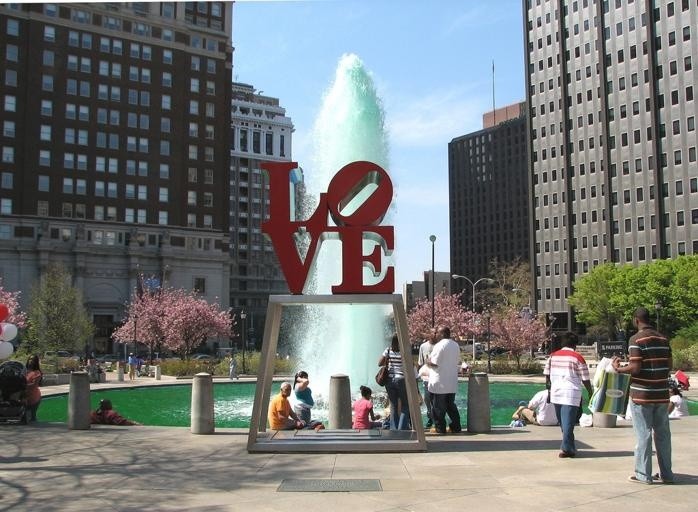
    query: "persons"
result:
[267,326,472,435]
[91,398,142,425]
[26,354,42,421]
[512,400,536,424]
[611,308,673,485]
[128,352,136,380]
[522,390,558,426]
[543,333,592,458]
[230,353,239,380]
[668,387,689,417]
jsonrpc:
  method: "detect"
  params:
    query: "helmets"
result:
[98,398,113,410]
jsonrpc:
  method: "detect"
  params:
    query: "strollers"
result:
[0,360,30,427]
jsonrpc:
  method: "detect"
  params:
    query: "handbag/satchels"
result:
[588,356,632,418]
[376,367,388,386]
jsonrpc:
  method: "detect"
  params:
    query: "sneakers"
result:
[652,472,675,485]
[630,475,654,485]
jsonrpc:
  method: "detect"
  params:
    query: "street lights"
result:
[130,312,143,379]
[453,272,495,371]
[428,233,440,344]
[237,305,253,380]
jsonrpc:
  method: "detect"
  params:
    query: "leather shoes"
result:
[559,451,575,458]
[446,427,462,433]
[430,427,446,433]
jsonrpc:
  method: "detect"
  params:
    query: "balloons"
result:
[0,303,17,359]
